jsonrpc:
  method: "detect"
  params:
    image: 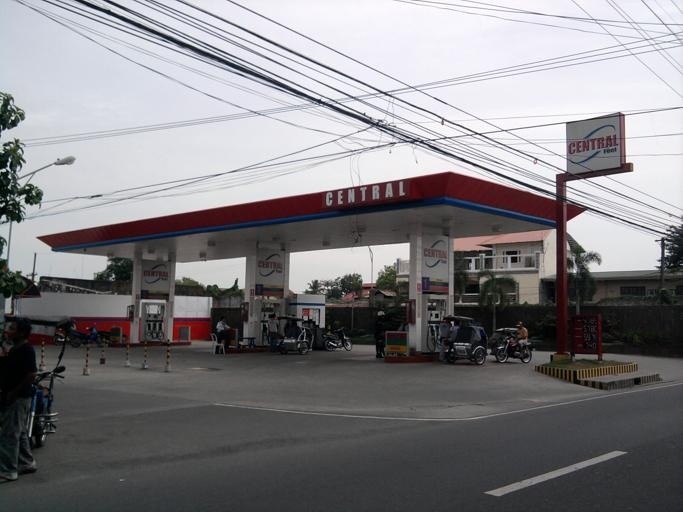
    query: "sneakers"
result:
[0,462,38,481]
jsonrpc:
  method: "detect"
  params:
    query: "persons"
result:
[438,318,452,362]
[515,321,529,359]
[268,313,279,352]
[374,310,386,358]
[215,315,230,354]
[442,320,460,347]
[0,315,38,480]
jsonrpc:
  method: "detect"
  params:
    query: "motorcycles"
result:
[67,323,101,348]
[53,319,77,345]
[443,315,488,366]
[24,364,66,447]
[274,315,314,354]
[489,326,532,363]
[321,323,353,352]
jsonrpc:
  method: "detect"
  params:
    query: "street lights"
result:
[3,154,76,267]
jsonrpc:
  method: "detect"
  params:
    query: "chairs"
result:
[209,333,225,355]
